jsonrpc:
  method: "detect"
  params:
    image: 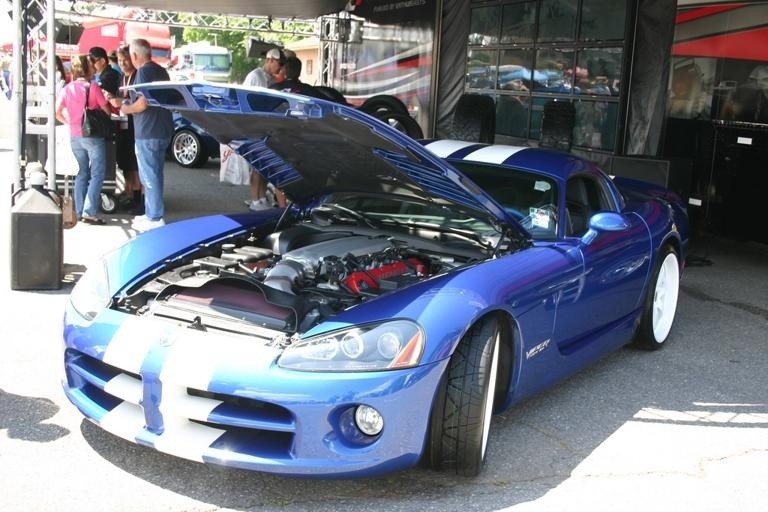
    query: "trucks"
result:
[74,21,172,79]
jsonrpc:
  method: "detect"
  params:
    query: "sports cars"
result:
[54,80,690,482]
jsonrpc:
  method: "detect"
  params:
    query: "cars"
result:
[166,113,220,167]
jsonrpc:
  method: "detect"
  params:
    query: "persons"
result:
[266,56,302,209]
[240,46,286,212]
[272,48,297,83]
[53,37,172,233]
[497,78,529,112]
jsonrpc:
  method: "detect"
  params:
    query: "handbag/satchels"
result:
[80,107,115,142]
[113,127,139,173]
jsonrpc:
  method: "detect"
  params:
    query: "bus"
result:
[173,41,234,84]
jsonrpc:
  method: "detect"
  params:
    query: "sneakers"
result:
[114,191,151,215]
[130,214,166,234]
[243,194,277,211]
[82,215,106,226]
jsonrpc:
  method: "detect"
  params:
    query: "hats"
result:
[86,46,120,61]
[264,47,288,65]
